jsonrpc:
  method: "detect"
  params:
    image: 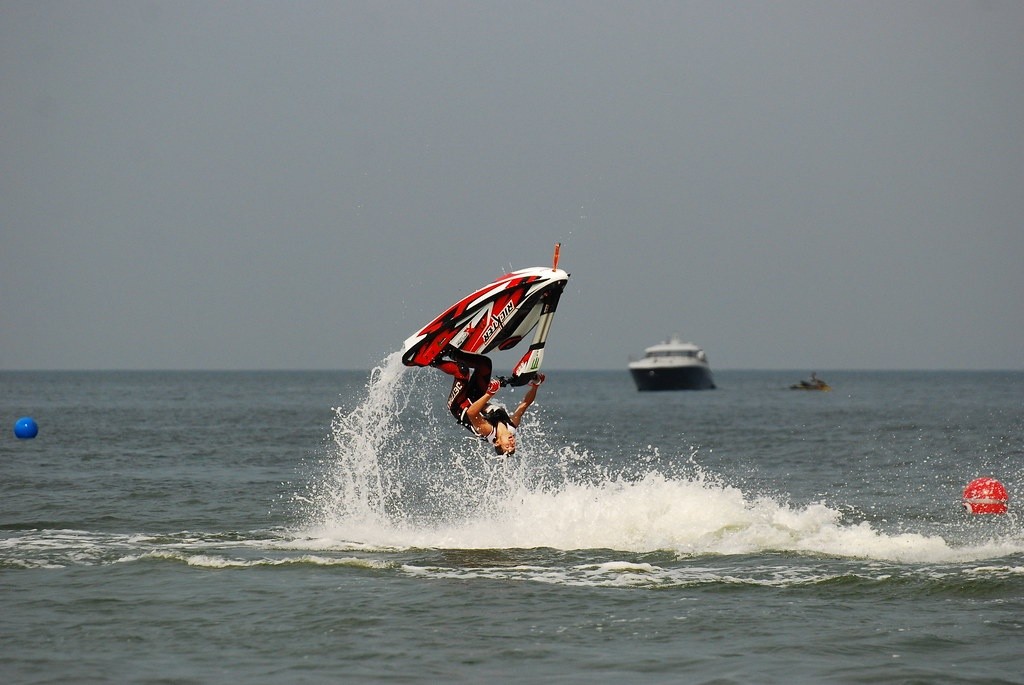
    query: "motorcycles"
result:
[789,379,833,392]
[401,241,572,390]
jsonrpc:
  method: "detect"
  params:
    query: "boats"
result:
[627,332,718,392]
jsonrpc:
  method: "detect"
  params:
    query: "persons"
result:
[431,346,545,457]
